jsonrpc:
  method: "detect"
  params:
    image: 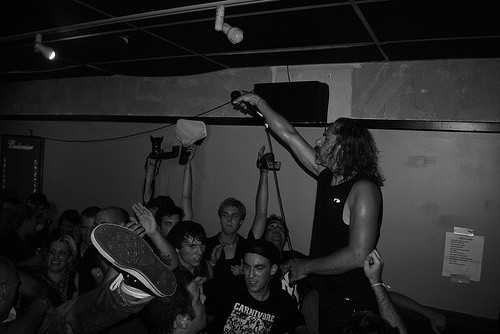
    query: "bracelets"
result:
[256,97,262,107]
[367,278,390,291]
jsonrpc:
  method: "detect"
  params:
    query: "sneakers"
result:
[90,223,177,298]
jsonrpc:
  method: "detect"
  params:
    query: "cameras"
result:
[148,135,179,159]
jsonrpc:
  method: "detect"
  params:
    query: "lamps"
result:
[33,33,56,60]
[214,5,244,44]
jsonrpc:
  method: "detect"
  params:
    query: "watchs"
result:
[32,296,51,308]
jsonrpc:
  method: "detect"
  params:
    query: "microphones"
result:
[230,90,263,118]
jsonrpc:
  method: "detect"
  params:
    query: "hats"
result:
[244,238,283,279]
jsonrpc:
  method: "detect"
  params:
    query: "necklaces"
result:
[218,233,237,246]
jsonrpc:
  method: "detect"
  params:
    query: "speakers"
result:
[254,81,329,122]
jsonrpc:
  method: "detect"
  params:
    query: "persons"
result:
[248,146,309,309]
[0,188,102,308]
[326,248,447,334]
[143,144,197,237]
[166,220,215,299]
[233,90,381,334]
[203,239,312,334]
[139,265,208,334]
[78,201,180,302]
[0,223,177,334]
[204,198,253,280]
